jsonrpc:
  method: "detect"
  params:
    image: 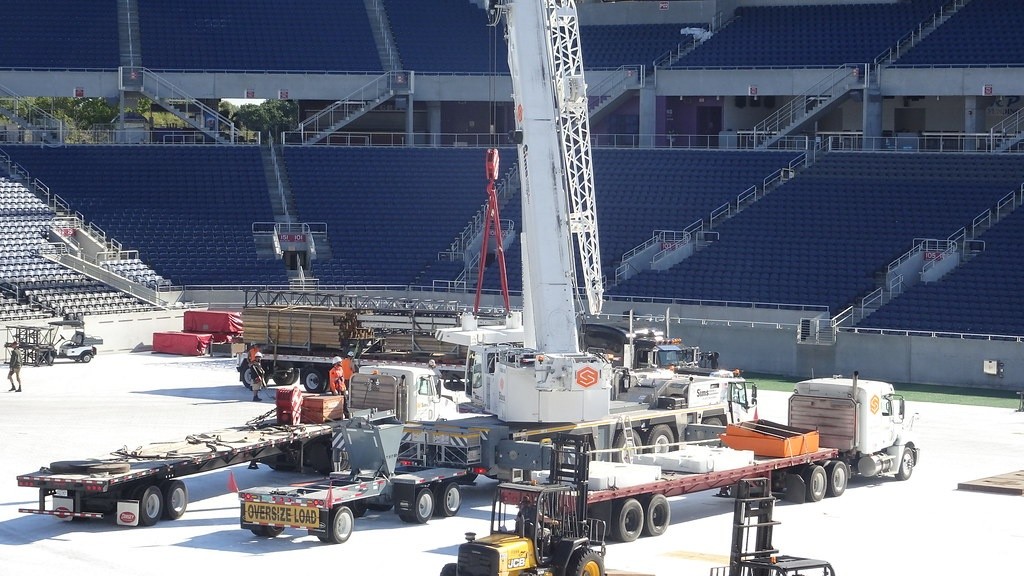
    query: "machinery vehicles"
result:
[440,432,608,576]
[422,1,758,481]
[711,477,836,576]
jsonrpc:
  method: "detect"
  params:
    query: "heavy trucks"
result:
[500,371,921,542]
[17,364,460,526]
[234,288,685,394]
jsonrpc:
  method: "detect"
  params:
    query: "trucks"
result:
[240,364,491,545]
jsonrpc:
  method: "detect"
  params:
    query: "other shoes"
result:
[9,387,15,391]
[253,397,262,401]
[16,389,21,392]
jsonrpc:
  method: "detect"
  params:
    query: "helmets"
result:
[12,341,19,347]
[347,351,355,357]
[255,352,262,357]
[429,360,435,365]
[333,356,342,364]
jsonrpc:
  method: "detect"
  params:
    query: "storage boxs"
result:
[299,393,343,423]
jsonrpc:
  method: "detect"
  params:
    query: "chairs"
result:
[537,358,566,388]
[0,144,1024,341]
[0,0,510,76]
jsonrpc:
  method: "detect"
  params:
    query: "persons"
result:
[7,341,23,392]
[329,351,355,419]
[428,359,443,398]
[519,495,559,551]
[246,341,265,402]
